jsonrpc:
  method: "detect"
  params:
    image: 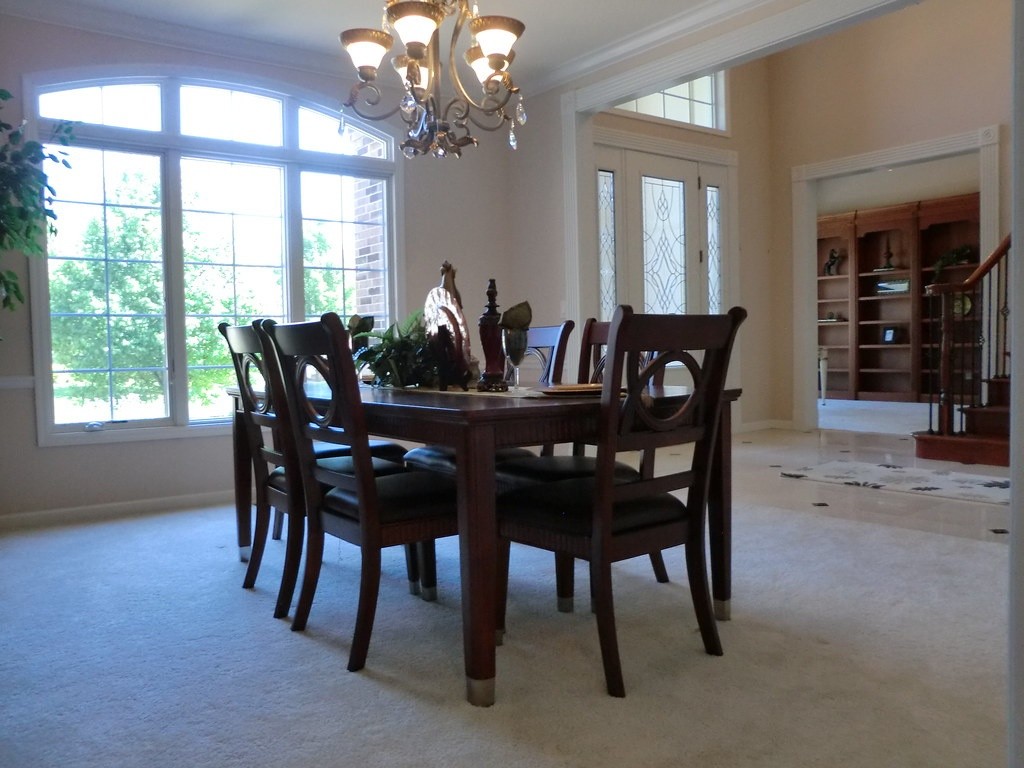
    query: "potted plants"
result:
[350,310,433,389]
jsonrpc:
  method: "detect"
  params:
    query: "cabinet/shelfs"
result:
[818,192,982,405]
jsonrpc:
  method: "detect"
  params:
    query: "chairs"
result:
[219,303,747,697]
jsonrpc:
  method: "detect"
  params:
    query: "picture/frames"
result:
[881,326,897,344]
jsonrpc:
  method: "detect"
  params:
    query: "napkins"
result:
[499,300,533,366]
[347,314,374,355]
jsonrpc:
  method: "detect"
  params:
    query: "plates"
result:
[540,383,603,396]
[952,295,972,316]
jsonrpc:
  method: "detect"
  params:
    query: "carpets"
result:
[780,459,1018,511]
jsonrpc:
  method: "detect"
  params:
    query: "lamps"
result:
[337,0,527,160]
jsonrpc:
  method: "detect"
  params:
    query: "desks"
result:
[226,381,742,706]
